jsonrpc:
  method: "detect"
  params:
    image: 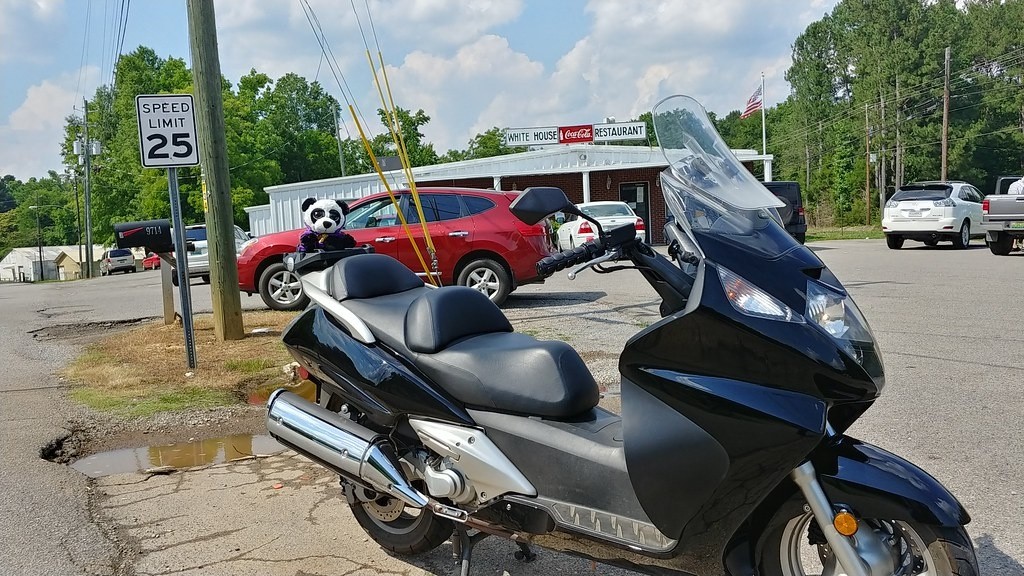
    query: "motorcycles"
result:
[263,92,980,576]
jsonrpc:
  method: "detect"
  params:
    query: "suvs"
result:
[172,222,256,285]
[881,179,986,249]
[98,248,136,276]
[690,180,806,245]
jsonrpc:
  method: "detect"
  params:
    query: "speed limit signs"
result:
[136,95,200,167]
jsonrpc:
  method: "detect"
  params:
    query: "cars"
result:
[237,185,556,301]
[141,252,161,270]
[554,201,645,249]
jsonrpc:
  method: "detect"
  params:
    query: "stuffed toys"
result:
[296,198,357,253]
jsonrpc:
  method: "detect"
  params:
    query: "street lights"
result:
[65,164,84,280]
[74,138,101,278]
[29,204,93,277]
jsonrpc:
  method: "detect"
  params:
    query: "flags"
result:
[739,83,763,120]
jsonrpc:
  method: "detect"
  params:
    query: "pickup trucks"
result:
[983,176,1024,254]
